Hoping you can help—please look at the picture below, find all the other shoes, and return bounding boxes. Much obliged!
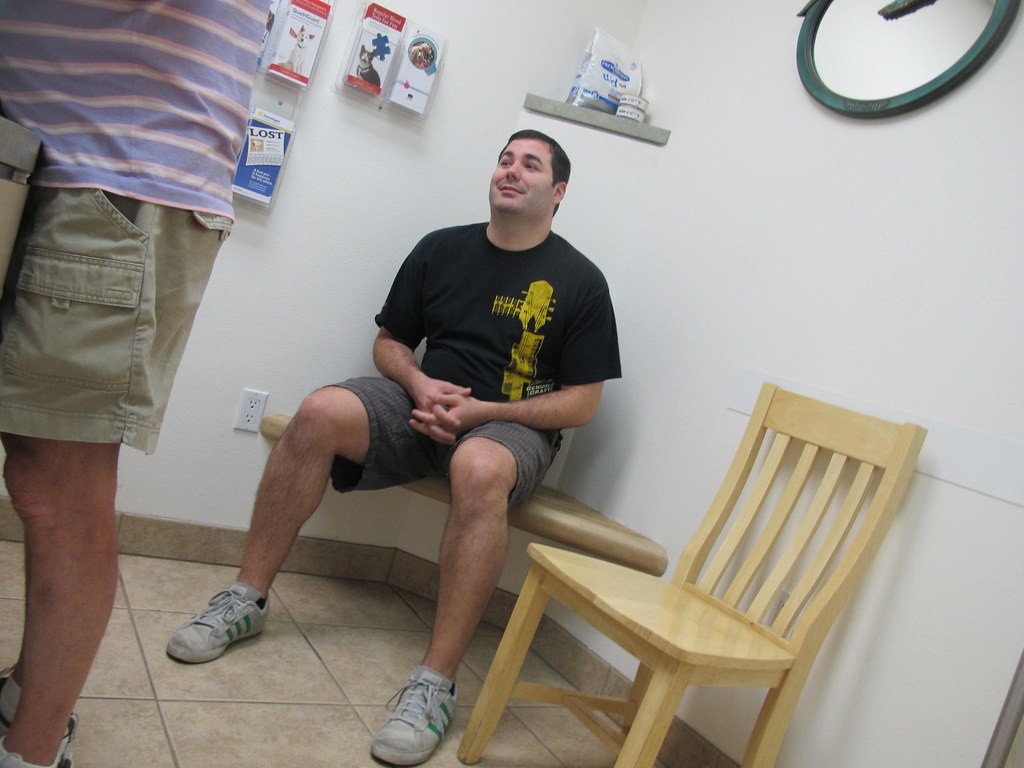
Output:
[0,664,78,768]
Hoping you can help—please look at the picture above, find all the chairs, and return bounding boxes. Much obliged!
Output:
[457,380,928,768]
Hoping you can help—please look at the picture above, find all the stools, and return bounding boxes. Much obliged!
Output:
[260,413,668,578]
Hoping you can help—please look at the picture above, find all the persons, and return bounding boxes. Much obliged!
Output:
[0,0,273,768]
[166,129,623,766]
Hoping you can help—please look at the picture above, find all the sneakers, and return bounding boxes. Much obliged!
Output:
[167,582,270,664]
[371,664,457,766]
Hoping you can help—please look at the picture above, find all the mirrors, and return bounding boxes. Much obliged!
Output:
[795,0,1021,120]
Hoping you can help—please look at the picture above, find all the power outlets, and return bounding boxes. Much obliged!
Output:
[233,388,269,433]
